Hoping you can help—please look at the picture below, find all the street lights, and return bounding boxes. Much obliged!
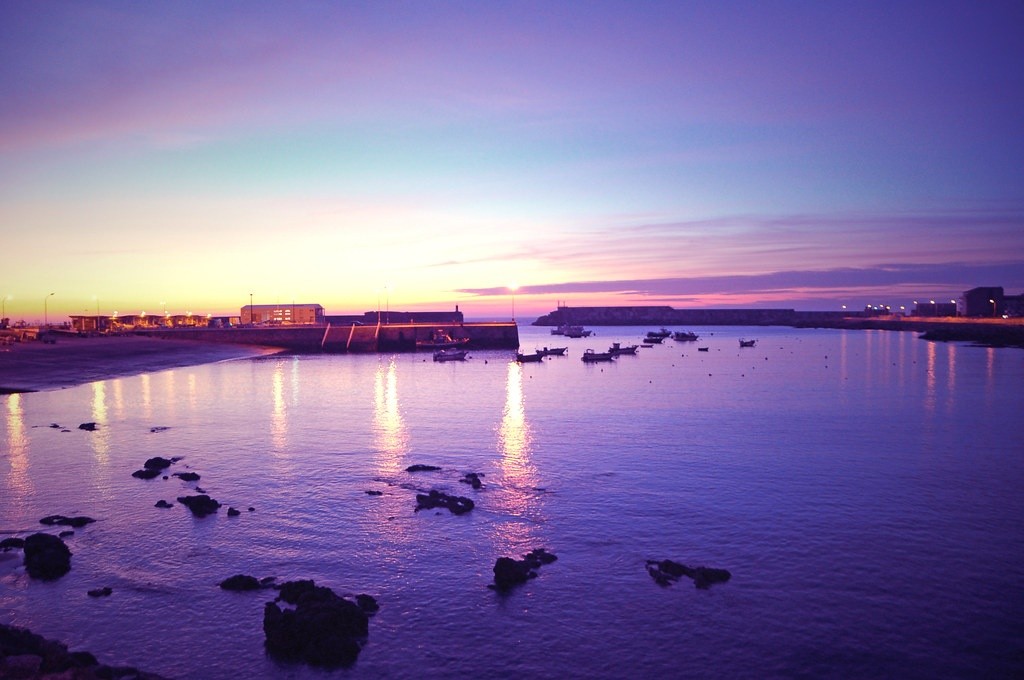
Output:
[250,294,253,323]
[44,292,56,325]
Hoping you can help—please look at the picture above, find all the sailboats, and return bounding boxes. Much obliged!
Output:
[550,301,584,335]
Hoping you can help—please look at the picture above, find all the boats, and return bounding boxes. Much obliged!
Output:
[581,327,710,362]
[416,330,471,351]
[739,338,756,347]
[535,346,568,355]
[432,348,468,362]
[564,328,593,339]
[517,353,544,362]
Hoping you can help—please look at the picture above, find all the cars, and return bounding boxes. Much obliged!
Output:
[254,322,268,328]
[350,320,364,326]
